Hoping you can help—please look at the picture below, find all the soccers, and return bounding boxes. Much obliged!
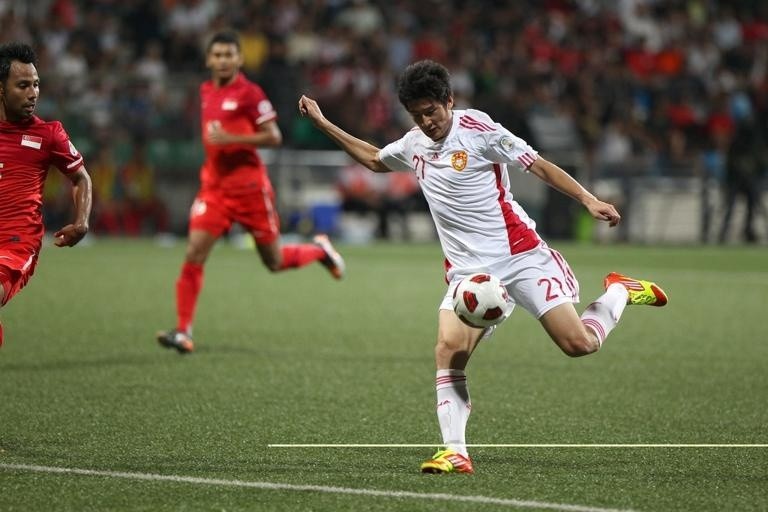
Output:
[453,273,508,328]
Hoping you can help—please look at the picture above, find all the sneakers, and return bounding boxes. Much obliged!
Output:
[420,448,474,473]
[602,270,668,308]
[157,328,195,354]
[313,233,347,280]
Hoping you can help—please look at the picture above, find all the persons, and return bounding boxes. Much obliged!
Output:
[154,31,347,353]
[297,59,669,473]
[0,42,93,346]
[1,0,766,248]
